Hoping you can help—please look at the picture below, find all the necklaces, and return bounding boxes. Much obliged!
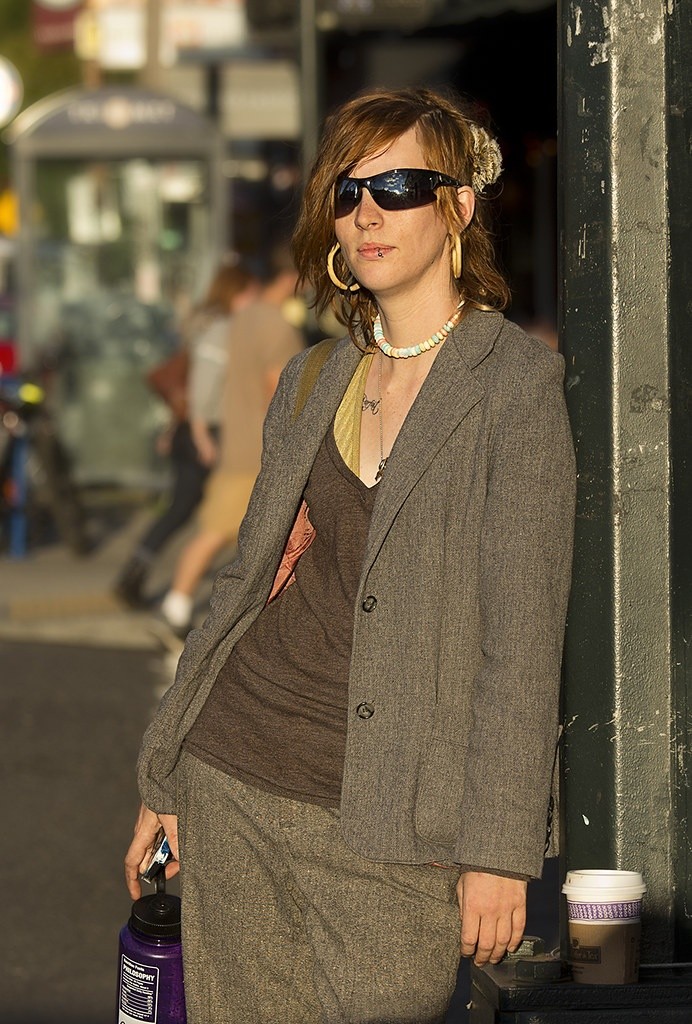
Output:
[372,304,462,358]
[374,351,389,481]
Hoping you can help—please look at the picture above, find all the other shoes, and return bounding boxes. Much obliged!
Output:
[107,580,163,613]
[153,605,196,654]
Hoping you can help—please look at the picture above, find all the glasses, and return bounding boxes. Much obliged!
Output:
[334,169,463,219]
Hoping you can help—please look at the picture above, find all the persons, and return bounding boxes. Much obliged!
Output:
[126,82,578,1024]
[102,246,308,648]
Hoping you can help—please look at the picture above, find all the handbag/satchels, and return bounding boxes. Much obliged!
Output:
[144,341,192,420]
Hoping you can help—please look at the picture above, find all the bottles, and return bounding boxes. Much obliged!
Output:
[117,862,187,1024]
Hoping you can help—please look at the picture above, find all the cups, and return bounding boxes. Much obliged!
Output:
[561,868,648,985]
